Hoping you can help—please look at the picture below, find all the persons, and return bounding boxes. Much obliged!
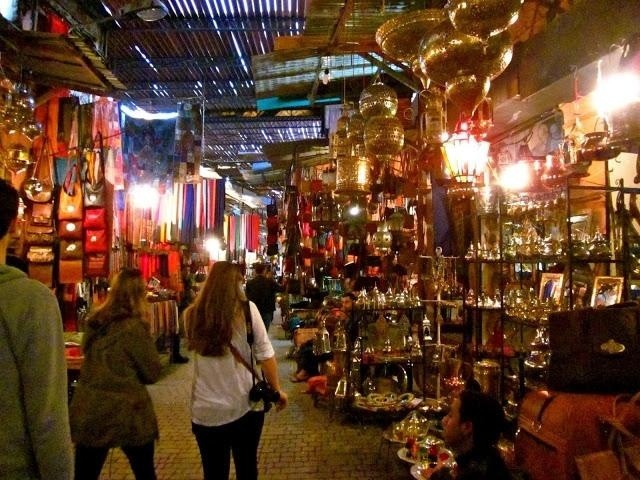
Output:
[65,266,163,479]
[0,177,75,480]
[246,263,288,333]
[288,292,357,382]
[168,260,193,367]
[440,387,517,480]
[180,260,287,480]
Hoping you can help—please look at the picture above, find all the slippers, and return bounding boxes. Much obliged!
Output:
[290,368,309,383]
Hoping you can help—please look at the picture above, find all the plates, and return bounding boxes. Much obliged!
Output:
[397,443,454,464]
[410,462,457,480]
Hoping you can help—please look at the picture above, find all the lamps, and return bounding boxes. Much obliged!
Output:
[412,89,454,147]
[67,0,168,34]
[338,153,371,193]
[419,22,515,116]
[358,71,399,115]
[445,0,548,56]
[360,112,405,169]
[329,113,361,157]
[376,8,449,64]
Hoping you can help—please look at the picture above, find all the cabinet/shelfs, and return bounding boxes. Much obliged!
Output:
[343,179,640,418]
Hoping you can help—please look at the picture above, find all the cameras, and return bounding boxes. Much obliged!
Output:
[250,380,280,405]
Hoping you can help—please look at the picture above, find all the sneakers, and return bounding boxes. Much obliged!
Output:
[170,356,189,364]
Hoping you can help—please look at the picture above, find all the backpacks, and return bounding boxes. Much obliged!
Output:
[59,260,84,285]
[512,388,605,480]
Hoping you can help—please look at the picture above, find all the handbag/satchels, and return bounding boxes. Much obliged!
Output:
[548,306,638,394]
[27,225,55,247]
[28,261,54,289]
[86,252,108,279]
[84,183,105,209]
[30,201,53,224]
[59,186,84,221]
[283,200,407,306]
[83,206,106,230]
[25,177,52,202]
[84,226,108,255]
[25,247,55,263]
[59,240,84,260]
[60,220,83,239]
